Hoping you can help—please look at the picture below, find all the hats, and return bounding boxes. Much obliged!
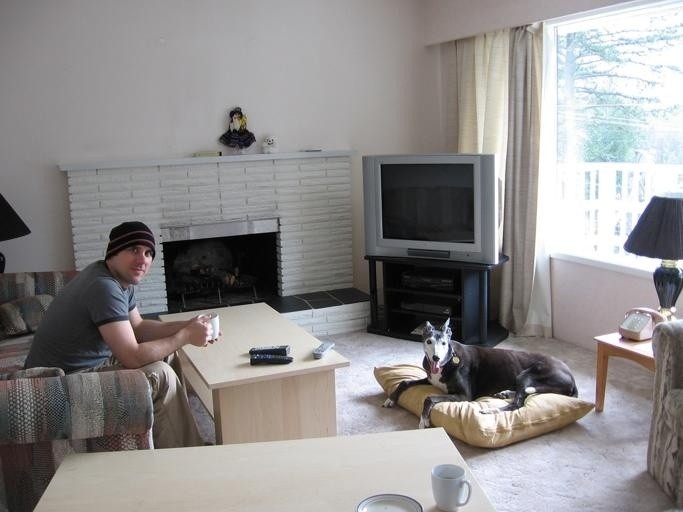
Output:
[105,221,156,263]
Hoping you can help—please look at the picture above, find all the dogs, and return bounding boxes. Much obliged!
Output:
[381,317,579,429]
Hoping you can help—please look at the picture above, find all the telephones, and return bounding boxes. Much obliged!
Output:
[618,307,668,342]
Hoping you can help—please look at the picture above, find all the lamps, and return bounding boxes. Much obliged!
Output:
[0,191,31,272]
[620,194,681,314]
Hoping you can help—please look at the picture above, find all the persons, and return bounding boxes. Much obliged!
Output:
[25,222,221,449]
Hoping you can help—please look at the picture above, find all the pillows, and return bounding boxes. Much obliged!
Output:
[372,358,595,448]
[1,366,65,379]
[0,292,56,338]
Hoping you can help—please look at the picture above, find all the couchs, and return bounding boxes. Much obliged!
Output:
[647,319,683,511]
[0,270,153,510]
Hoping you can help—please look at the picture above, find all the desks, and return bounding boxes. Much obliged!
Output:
[592,314,654,412]
[33,425,494,510]
[153,301,351,444]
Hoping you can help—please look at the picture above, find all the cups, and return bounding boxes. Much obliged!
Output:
[198,312,220,340]
[431,463,472,510]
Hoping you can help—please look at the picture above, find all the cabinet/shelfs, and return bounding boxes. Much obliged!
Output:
[365,258,511,350]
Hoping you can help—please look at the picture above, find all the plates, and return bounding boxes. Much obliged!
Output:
[357,494,425,512]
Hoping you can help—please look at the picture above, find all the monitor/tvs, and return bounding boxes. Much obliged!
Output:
[361,152,502,266]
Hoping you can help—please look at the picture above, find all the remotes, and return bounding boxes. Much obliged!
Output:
[250,353,293,365]
[312,341,335,359]
[249,344,290,356]
